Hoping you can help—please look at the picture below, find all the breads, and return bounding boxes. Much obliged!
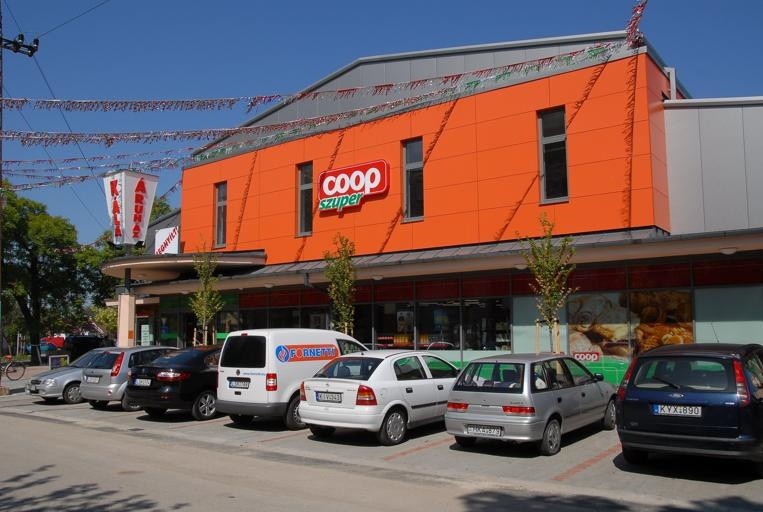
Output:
[568,294,641,357]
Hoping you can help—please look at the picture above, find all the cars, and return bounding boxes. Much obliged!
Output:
[25,347,116,405]
[122,343,223,421]
[297,349,488,446]
[79,346,181,412]
[445,352,620,456]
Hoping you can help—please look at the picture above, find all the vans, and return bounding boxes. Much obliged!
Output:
[214,328,375,431]
[614,343,763,475]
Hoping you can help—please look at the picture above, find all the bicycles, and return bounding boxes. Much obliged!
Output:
[0,354,28,382]
